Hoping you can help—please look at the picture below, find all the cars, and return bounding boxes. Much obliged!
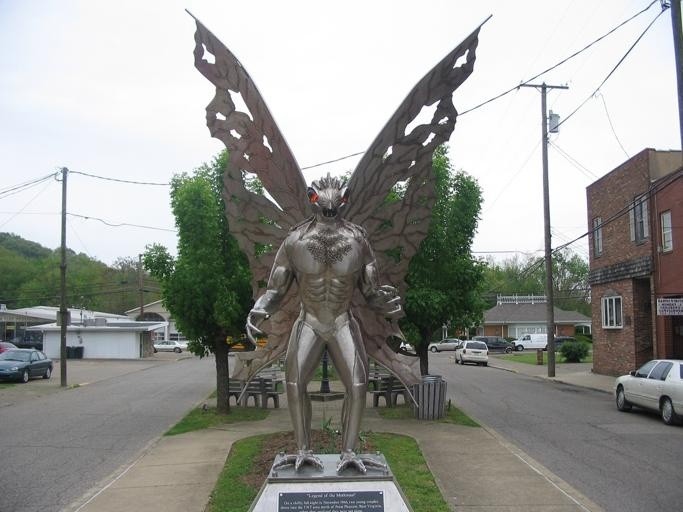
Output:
[0,347,51,382]
[400,342,418,357]
[427,337,463,353]
[0,340,16,357]
[551,335,581,344]
[9,337,43,351]
[153,339,189,354]
[455,337,488,366]
[615,358,682,424]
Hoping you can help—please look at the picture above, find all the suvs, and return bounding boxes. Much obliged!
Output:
[472,335,515,355]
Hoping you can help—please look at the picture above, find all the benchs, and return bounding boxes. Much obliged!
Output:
[370,376,413,407]
[229,378,282,409]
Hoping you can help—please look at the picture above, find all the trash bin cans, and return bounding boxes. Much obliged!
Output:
[537,348,543,365]
[65,346,84,359]
[413,374,447,420]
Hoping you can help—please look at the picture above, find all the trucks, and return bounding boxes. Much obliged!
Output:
[511,333,556,352]
